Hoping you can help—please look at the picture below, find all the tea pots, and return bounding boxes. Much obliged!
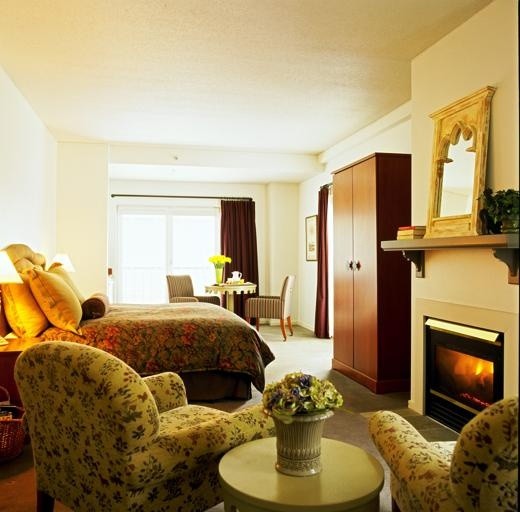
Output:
[232,271,243,280]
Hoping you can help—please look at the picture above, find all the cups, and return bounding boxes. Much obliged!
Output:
[226,277,245,286]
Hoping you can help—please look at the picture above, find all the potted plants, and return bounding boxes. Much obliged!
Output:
[476,186,520,233]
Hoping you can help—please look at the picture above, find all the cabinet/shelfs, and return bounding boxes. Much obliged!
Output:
[331,152,410,393]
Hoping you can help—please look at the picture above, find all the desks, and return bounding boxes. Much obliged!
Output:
[203,281,257,312]
[216,434,385,512]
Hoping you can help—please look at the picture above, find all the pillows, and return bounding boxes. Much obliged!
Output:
[1,244,111,341]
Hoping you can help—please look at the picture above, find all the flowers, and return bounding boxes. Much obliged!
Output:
[263,368,346,425]
[208,255,232,269]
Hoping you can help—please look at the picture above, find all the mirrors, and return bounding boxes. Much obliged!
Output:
[424,86,498,238]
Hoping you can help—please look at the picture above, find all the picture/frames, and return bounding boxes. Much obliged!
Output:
[305,215,319,262]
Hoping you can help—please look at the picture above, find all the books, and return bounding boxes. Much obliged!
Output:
[396,225,426,240]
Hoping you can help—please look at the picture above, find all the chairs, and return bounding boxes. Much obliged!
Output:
[369,394,519,511]
[166,273,221,306]
[245,274,295,341]
[13,341,276,512]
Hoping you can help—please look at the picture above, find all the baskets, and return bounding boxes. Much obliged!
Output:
[0,405,26,464]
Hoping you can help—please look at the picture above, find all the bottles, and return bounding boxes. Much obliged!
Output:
[107,268,115,305]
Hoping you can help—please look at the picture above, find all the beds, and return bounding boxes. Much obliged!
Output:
[0,280,275,403]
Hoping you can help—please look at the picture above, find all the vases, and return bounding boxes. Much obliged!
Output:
[214,264,224,282]
[269,408,334,478]
